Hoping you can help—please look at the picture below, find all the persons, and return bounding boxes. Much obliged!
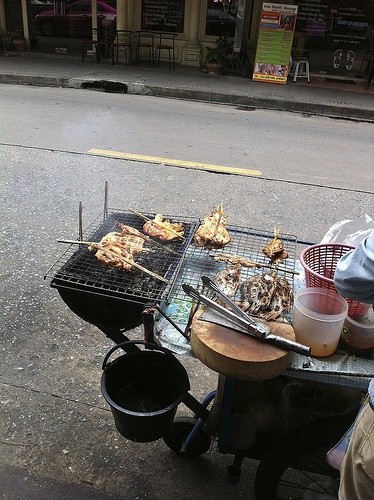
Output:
[333,228,373,500]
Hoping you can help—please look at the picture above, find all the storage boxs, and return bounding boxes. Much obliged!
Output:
[304,29,326,38]
[304,19,327,30]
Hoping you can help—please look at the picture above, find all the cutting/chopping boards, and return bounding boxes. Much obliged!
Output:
[189,300,296,383]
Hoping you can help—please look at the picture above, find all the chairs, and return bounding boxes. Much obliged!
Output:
[81,27,100,65]
[154,32,178,72]
[134,30,155,72]
[110,29,134,68]
[96,28,116,66]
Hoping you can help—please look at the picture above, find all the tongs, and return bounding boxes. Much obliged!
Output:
[182,274,267,338]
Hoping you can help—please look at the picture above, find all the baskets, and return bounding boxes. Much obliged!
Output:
[300,243,371,317]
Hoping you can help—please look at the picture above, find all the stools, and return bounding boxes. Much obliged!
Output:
[291,57,310,83]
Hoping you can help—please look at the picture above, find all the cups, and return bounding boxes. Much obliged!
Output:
[343,303,374,350]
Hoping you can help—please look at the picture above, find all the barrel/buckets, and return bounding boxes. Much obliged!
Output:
[293,288,349,357]
[101,340,190,444]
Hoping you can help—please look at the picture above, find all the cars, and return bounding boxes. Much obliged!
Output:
[32,0,117,37]
[160,8,237,37]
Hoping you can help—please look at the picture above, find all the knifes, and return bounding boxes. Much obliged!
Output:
[197,305,311,358]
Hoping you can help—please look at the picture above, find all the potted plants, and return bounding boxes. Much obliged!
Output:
[29,31,40,50]
[205,35,234,76]
[11,30,27,50]
